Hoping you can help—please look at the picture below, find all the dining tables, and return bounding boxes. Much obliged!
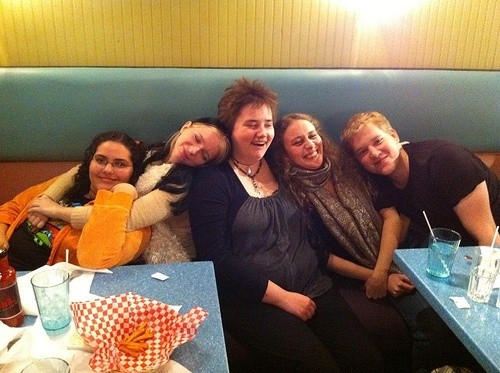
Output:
[14,262,230,373]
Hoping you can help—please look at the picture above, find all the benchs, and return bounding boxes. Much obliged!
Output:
[0,67,500,280]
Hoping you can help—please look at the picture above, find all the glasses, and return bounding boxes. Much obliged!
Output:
[92,153,134,169]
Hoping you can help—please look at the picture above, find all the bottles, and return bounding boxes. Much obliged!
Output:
[0,247,25,327]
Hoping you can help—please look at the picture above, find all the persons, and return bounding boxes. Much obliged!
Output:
[0,77,500,373]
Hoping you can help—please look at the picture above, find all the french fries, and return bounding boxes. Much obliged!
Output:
[116,323,154,357]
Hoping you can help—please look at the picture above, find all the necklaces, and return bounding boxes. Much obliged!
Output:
[230,155,263,188]
[233,157,258,173]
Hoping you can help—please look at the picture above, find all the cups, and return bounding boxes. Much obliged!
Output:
[468,246,500,304]
[21,357,70,373]
[426,227,461,278]
[30,268,72,335]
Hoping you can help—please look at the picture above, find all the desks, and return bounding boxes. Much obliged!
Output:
[393,246,500,373]
